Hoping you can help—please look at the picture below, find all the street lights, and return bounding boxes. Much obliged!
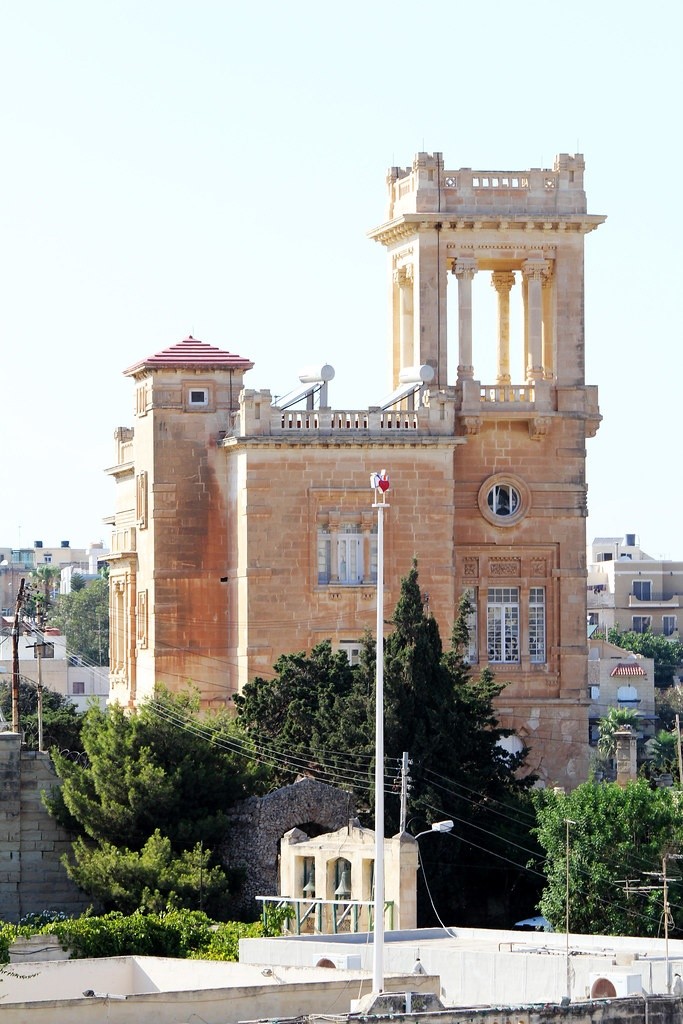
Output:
[350,467,391,1012]
[0,665,44,752]
